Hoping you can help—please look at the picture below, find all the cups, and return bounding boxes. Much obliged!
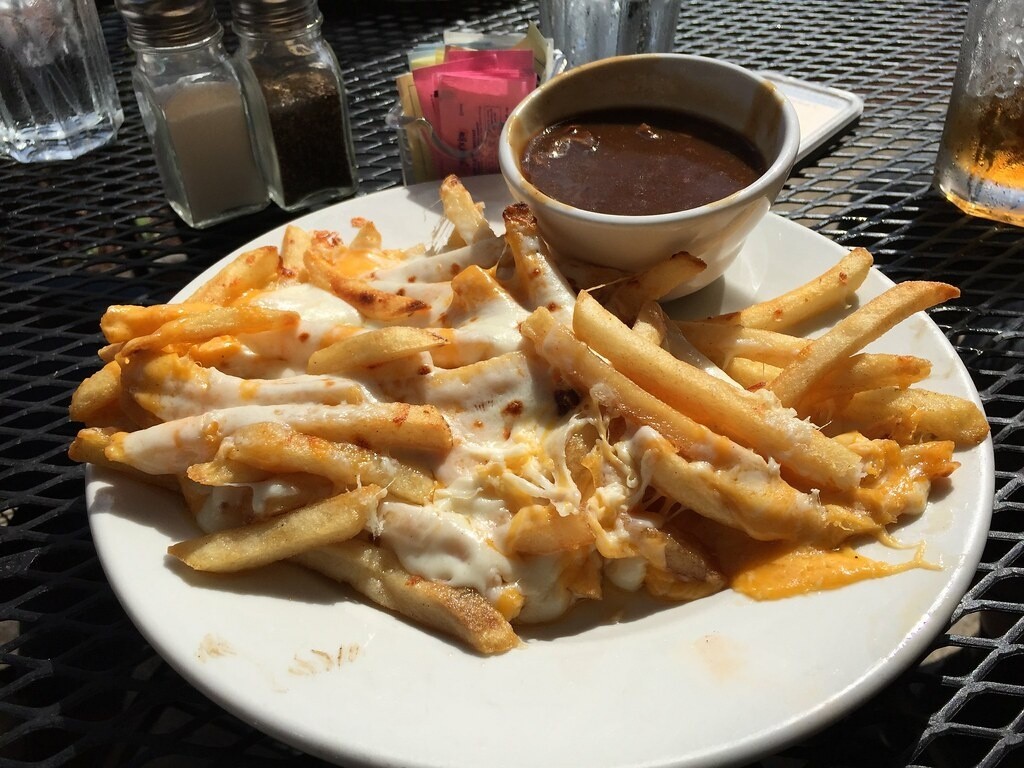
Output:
[934,0,1024,228]
[1,0,125,163]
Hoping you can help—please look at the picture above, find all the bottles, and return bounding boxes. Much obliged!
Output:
[230,0,357,213]
[112,0,272,226]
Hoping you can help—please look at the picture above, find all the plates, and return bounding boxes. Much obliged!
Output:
[85,170,991,768]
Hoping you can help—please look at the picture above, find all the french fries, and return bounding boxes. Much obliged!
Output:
[69,170,988,659]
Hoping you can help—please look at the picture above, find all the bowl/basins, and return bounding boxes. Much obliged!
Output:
[500,53,800,300]
